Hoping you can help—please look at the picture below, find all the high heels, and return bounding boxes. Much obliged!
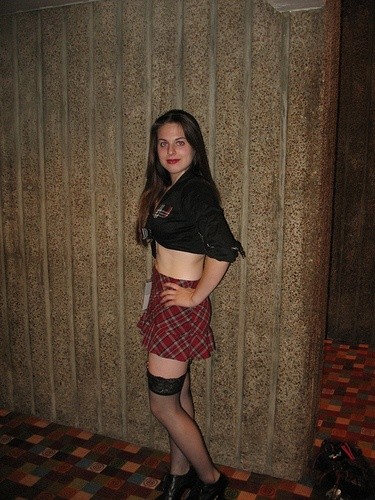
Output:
[155,467,197,500]
[187,474,229,499]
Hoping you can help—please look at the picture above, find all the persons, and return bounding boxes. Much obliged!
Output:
[134,110,247,499]
